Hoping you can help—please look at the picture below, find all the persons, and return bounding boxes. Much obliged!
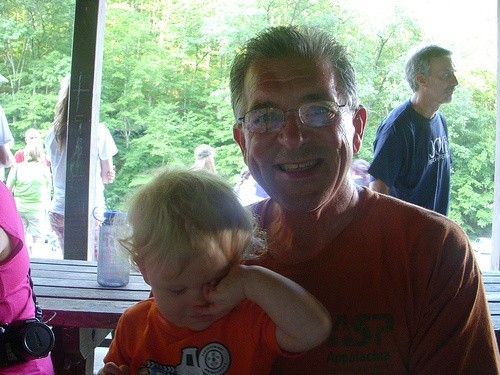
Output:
[0,72,117,374]
[368,46,459,215]
[101,169,333,375]
[226,26,500,375]
[190,144,216,173]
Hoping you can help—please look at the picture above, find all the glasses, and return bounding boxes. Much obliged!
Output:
[237,100,353,134]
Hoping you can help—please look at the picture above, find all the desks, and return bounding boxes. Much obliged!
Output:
[29,258,500,375]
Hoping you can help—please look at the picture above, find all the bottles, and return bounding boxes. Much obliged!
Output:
[93,205,132,287]
